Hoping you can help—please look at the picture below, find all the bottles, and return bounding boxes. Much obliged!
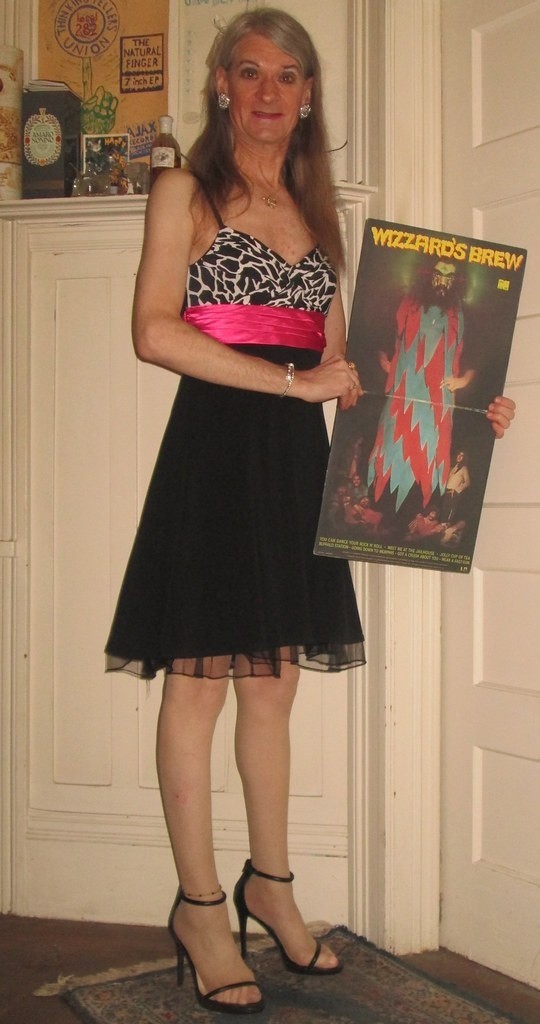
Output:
[151,116,181,194]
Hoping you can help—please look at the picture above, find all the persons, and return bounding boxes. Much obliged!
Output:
[106,8,515,1014]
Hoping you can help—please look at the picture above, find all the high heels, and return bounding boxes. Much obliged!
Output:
[233,859,342,975]
[168,885,266,1014]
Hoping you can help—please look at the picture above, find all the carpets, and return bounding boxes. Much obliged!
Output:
[34,921,531,1024]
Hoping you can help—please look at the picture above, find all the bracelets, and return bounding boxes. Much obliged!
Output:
[280,362,295,398]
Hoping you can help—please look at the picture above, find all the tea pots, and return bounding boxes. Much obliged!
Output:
[69,158,110,196]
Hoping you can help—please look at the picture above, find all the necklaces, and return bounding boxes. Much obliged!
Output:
[252,191,280,208]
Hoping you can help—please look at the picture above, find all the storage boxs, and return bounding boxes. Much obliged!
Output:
[21,91,81,199]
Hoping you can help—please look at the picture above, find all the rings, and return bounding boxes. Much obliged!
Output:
[347,360,355,370]
